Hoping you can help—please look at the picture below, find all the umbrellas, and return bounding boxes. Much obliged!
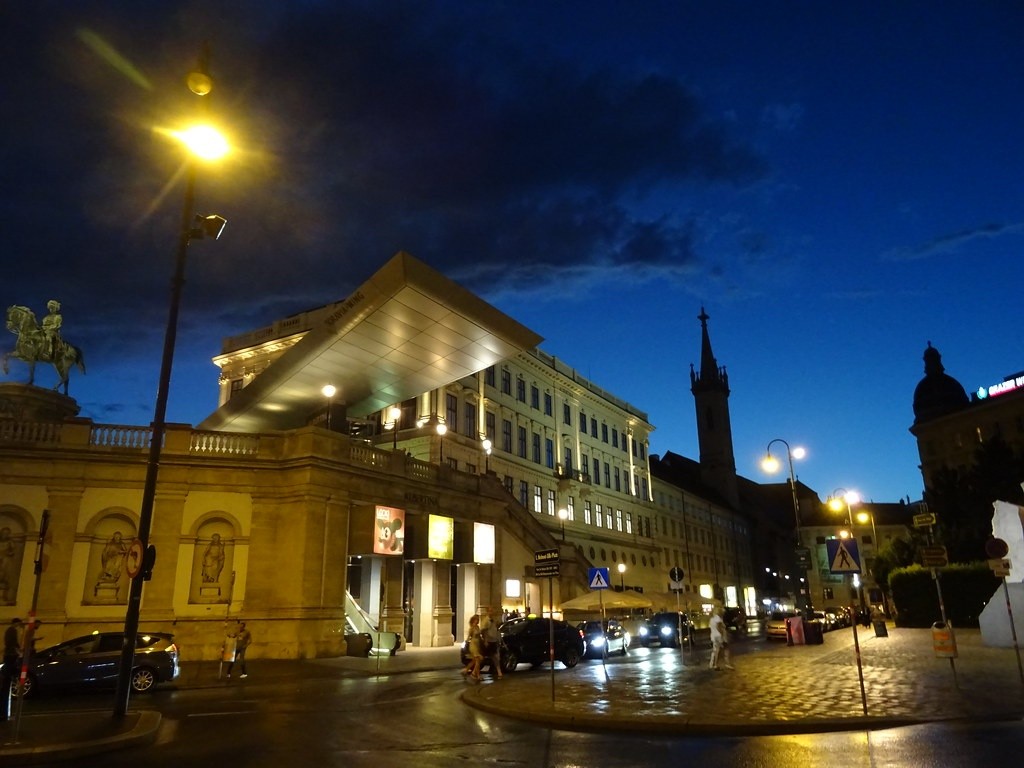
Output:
[557,588,721,655]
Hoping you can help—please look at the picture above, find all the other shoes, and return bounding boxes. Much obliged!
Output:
[714,667,722,671]
[498,673,503,676]
[460,670,466,680]
[239,674,247,679]
[726,665,734,670]
[226,674,230,676]
[470,673,477,678]
[475,677,484,681]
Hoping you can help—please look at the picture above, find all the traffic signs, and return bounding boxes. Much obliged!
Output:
[913,512,936,526]
[921,556,948,568]
[920,545,947,557]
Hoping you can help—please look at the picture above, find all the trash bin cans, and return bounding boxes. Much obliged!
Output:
[803,622,824,644]
[873,620,887,636]
[931,621,958,658]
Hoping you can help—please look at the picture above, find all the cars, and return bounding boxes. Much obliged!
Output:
[639,611,696,647]
[765,608,802,640]
[577,620,633,659]
[804,602,886,633]
[0,632,182,700]
[461,616,587,673]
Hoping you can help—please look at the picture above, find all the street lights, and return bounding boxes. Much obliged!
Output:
[761,439,815,622]
[854,511,886,614]
[109,44,229,736]
[828,487,868,623]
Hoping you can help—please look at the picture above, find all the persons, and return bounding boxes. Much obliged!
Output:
[861,604,896,629]
[41,300,62,357]
[648,608,734,671]
[0,527,16,604]
[99,531,128,582]
[4,618,21,659]
[201,533,225,583]
[225,623,252,679]
[26,620,44,655]
[459,607,531,681]
